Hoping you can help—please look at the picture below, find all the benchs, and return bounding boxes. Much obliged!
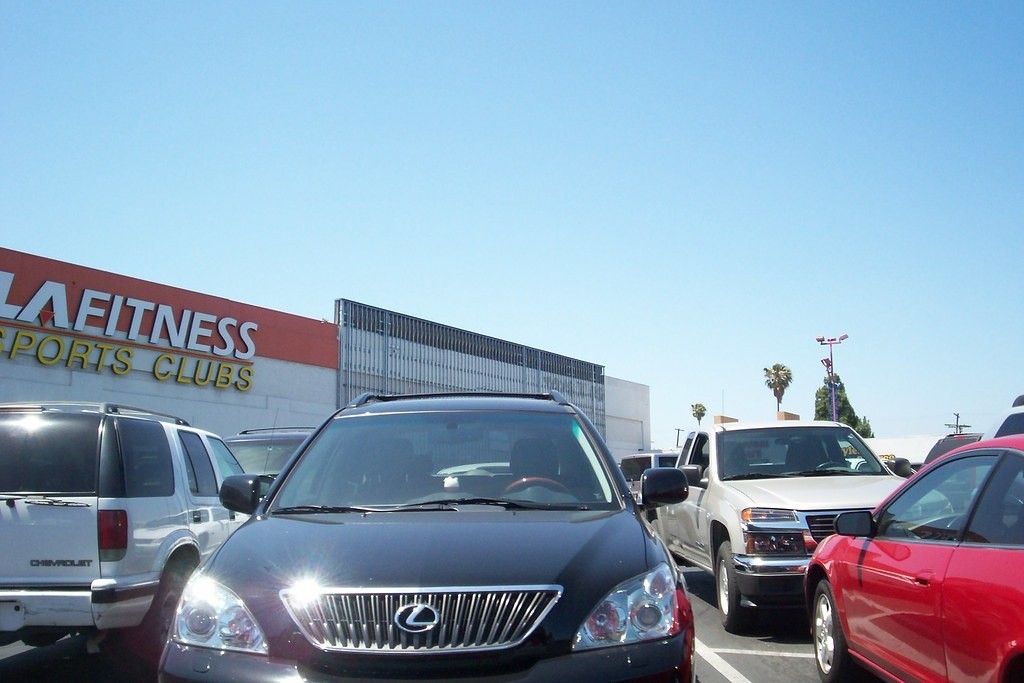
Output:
[432,474,512,497]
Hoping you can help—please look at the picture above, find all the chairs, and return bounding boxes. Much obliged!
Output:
[783,436,828,472]
[356,434,422,503]
[723,441,751,477]
[509,436,561,490]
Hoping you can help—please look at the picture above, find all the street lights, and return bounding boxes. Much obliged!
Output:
[816,332,849,420]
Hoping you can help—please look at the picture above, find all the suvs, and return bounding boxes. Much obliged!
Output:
[221,427,318,475]
[0,403,247,683]
[158,389,1024,683]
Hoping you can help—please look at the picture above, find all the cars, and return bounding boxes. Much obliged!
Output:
[804,434,1023,682]
